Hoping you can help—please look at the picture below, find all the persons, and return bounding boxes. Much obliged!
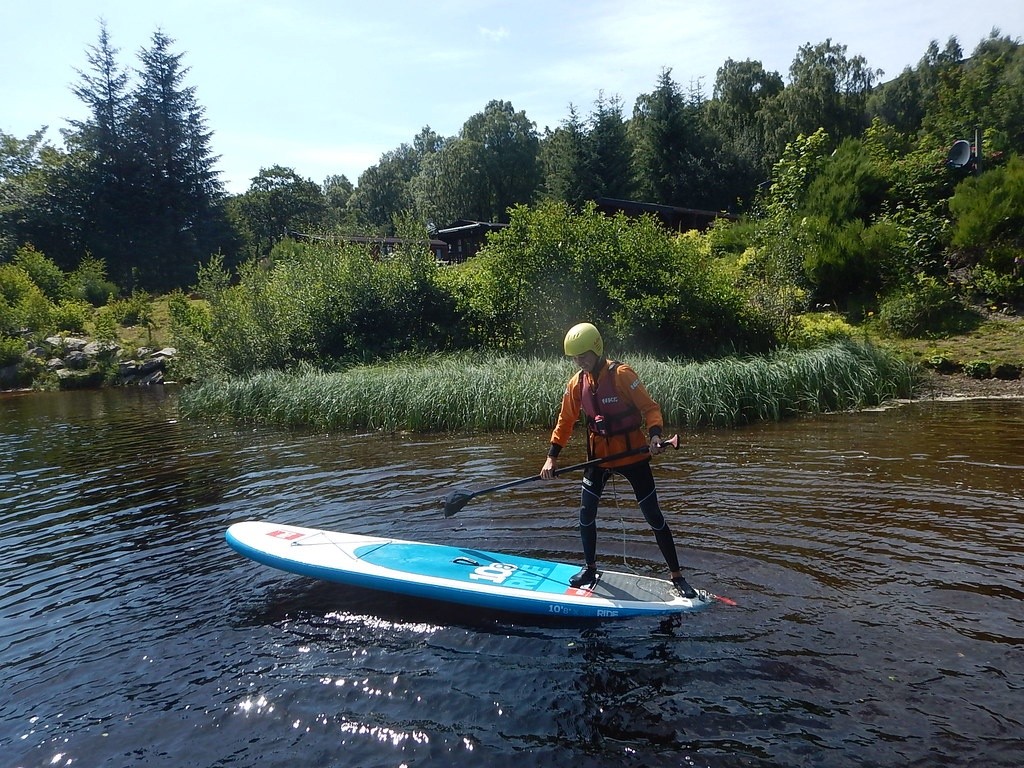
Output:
[539,322,698,598]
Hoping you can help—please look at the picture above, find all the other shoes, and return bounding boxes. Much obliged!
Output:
[671,577,695,598]
[570,566,597,586]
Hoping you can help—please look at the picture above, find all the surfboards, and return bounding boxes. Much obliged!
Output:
[224,518,712,615]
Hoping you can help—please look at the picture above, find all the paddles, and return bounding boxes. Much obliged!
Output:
[444,434,680,516]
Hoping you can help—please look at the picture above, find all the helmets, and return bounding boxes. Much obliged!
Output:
[564,323,603,358]
